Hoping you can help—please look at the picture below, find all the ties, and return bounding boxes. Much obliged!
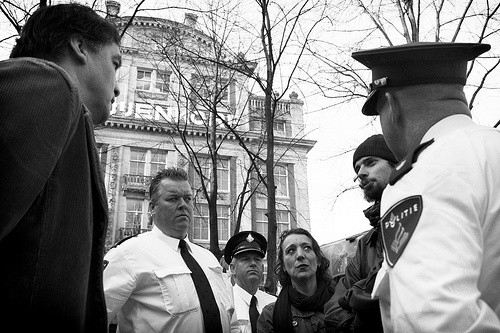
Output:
[249,295,260,332]
[177,239,223,333]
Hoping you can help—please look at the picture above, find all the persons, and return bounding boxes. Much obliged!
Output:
[351,41,500,333]
[103,167,242,333]
[257,227,336,333]
[324,132,400,333]
[0,2,122,333]
[224,230,278,332]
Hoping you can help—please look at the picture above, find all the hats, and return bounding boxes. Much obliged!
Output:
[351,42,491,116]
[224,230,267,264]
[353,134,398,167]
